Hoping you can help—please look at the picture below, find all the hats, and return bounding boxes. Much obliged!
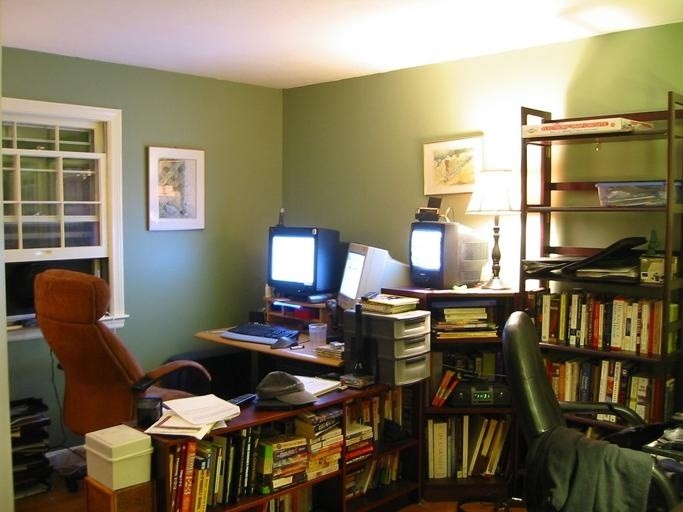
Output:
[256,371,320,405]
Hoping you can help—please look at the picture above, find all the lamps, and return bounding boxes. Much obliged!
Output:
[464,167,520,289]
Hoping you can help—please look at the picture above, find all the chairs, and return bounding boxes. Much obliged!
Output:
[33,268,212,434]
[500,311,682,512]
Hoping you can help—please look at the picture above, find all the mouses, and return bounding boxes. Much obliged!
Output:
[270,337,296,349]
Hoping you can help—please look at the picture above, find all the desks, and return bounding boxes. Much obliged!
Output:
[191,296,347,369]
[641,421,682,460]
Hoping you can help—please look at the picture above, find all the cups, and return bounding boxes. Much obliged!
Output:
[309,322,328,351]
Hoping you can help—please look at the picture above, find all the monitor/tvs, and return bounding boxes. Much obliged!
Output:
[411,221,492,288]
[338,240,409,306]
[265,225,348,298]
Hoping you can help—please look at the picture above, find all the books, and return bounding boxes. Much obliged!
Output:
[315,344,344,358]
[528,288,682,427]
[162,373,415,510]
[357,293,420,314]
[424,298,511,479]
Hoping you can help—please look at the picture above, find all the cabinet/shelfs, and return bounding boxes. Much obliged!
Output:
[381,287,547,503]
[520,91,682,444]
[135,373,423,512]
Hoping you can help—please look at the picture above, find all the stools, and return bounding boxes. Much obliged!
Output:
[82,476,157,512]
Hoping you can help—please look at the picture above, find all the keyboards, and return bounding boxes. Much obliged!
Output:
[219,320,300,346]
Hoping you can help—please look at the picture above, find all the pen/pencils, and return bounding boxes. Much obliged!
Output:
[290,345,304,350]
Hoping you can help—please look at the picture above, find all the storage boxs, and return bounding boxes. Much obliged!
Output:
[343,308,431,385]
[85,424,154,491]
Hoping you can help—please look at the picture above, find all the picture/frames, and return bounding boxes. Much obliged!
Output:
[422,131,484,196]
[146,144,207,232]
